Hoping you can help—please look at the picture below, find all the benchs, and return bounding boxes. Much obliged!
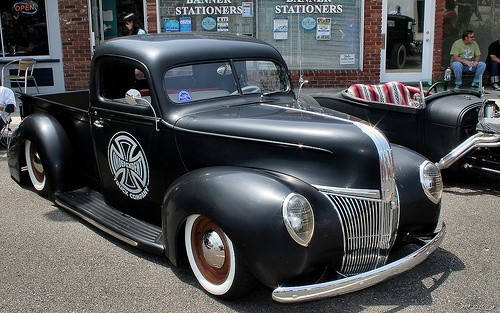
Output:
[348,81,414,107]
[140,87,220,95]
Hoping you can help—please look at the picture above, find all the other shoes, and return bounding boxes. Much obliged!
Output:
[471,82,484,89]
[455,84,461,89]
[491,83,500,90]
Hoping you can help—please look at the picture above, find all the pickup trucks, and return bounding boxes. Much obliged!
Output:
[7,32,446,302]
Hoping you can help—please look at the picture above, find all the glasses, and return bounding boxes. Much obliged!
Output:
[467,36,474,38]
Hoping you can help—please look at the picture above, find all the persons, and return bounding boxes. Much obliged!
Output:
[25,24,42,55]
[485,39,500,91]
[450,30,486,90]
[0,86,17,131]
[121,11,146,79]
[389,5,402,15]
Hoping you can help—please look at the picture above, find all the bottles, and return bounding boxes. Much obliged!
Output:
[444,67,452,81]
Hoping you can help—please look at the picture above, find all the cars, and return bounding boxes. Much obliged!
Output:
[307,80,500,177]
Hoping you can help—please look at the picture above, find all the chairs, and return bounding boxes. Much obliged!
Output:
[0,113,11,147]
[2,57,40,95]
